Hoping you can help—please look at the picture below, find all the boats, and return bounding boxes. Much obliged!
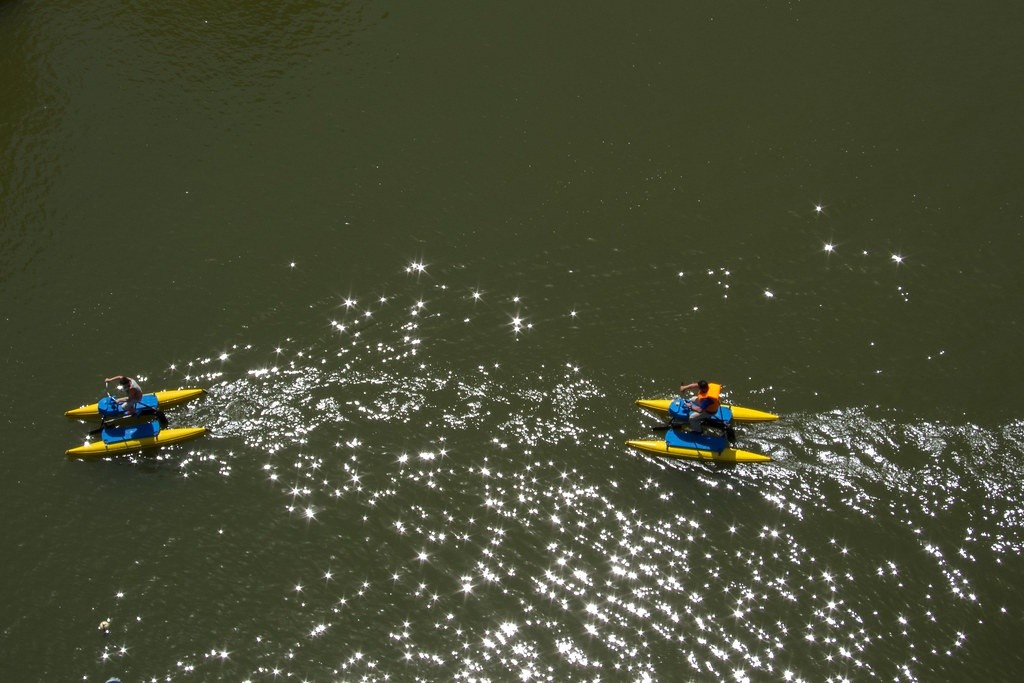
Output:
[624,382,784,464]
[64,378,210,458]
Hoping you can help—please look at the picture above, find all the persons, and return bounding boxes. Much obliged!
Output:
[681,380,721,432]
[105,376,142,411]
